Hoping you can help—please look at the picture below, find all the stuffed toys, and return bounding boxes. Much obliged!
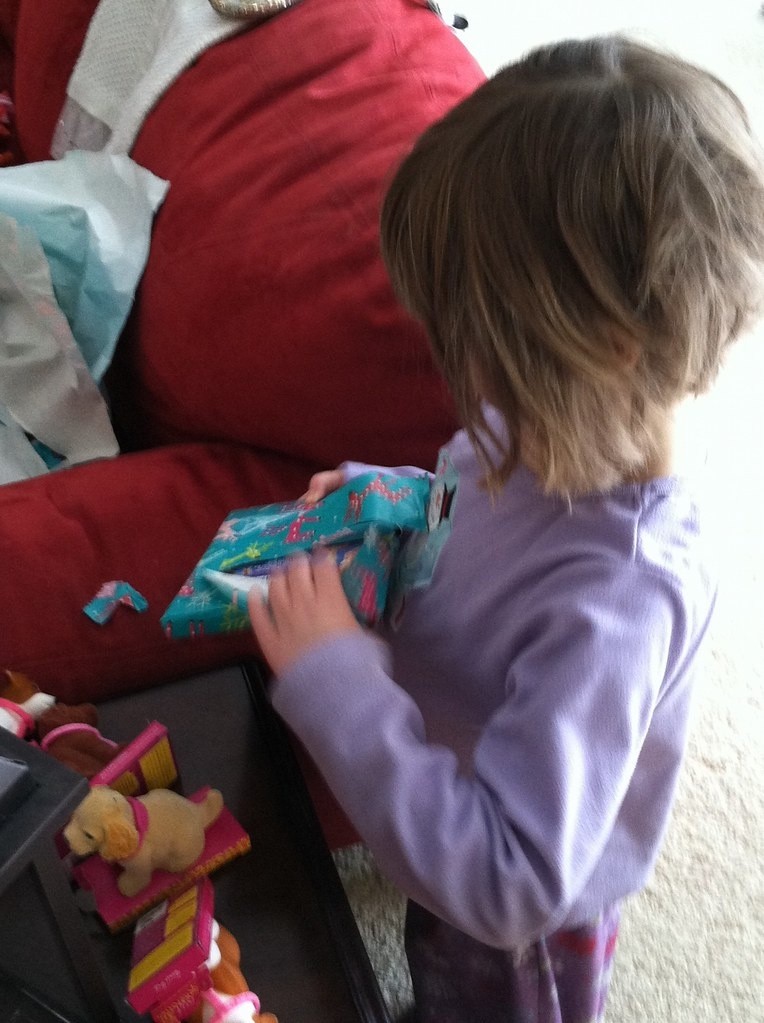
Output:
[202,919,278,1023]
[0,670,101,777]
[65,785,225,893]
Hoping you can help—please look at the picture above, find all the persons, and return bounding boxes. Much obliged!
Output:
[247,38,764,1023]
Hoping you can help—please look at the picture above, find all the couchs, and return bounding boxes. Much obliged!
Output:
[0,0,482,711]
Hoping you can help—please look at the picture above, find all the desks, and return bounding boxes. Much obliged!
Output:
[0,664,392,1023]
